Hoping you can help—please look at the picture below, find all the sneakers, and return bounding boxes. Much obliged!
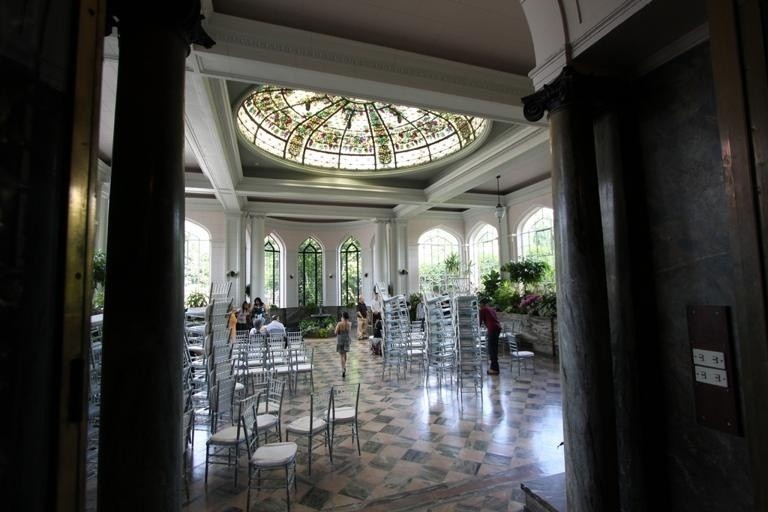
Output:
[487,369,498,374]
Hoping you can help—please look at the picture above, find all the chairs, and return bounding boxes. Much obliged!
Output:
[369,280,521,401]
[505,332,536,375]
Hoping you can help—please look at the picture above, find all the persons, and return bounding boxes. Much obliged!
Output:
[333,311,353,378]
[439,293,451,316]
[478,298,502,375]
[399,294,412,314]
[371,292,381,328]
[416,297,426,333]
[356,295,370,341]
[226,296,288,350]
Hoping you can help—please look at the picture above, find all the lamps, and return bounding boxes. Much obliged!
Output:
[494,176,506,225]
